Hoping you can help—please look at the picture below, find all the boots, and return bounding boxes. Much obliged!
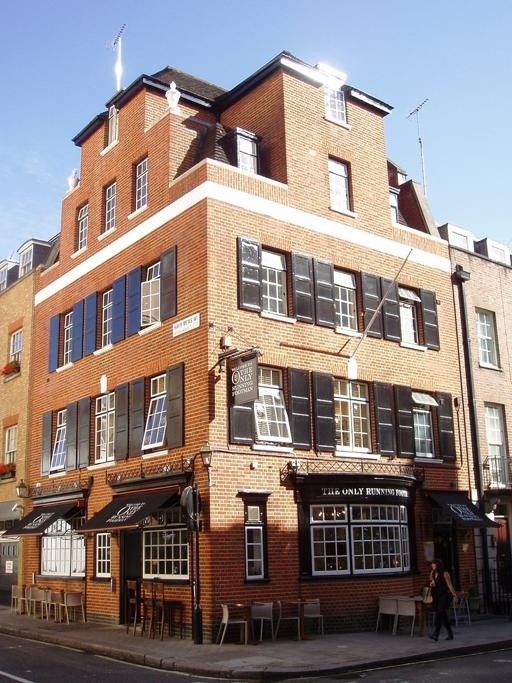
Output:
[428,632,454,641]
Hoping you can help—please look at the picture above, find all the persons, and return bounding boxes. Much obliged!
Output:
[429,559,457,641]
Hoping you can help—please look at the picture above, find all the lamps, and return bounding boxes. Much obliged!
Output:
[16,478,28,498]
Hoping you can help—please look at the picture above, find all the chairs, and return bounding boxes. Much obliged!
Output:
[126,579,183,640]
[11,584,86,625]
[214,598,324,646]
[375,594,471,638]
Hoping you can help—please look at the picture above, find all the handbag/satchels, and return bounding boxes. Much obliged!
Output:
[423,587,433,603]
